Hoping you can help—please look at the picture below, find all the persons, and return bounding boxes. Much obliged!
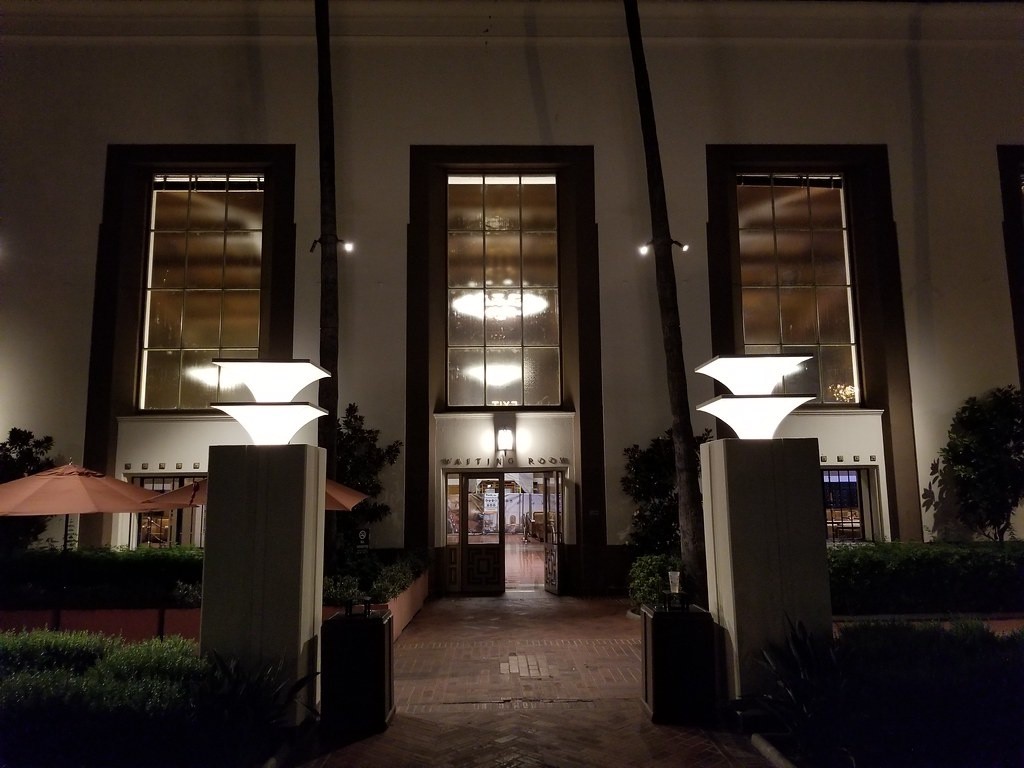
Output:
[522,511,531,534]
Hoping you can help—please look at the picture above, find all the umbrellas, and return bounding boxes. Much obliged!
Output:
[0,458,199,550]
[140,478,370,512]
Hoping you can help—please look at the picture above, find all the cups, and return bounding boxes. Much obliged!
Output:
[669,571,680,593]
